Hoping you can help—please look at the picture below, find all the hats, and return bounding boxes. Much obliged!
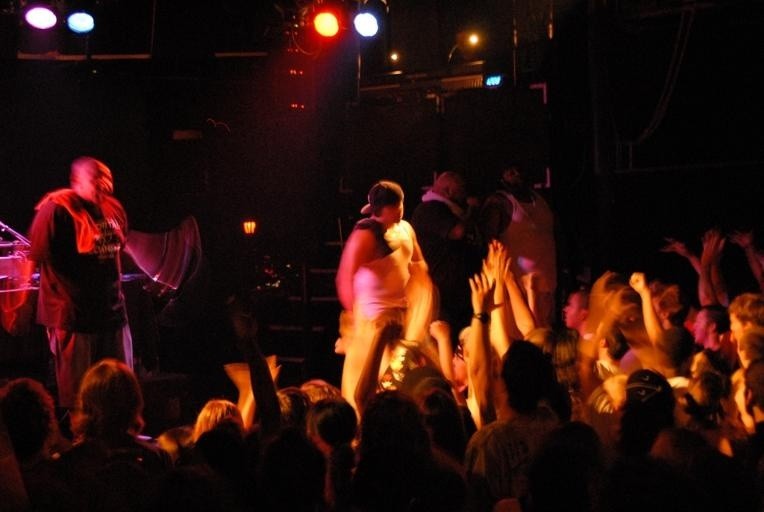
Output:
[360,181,404,215]
